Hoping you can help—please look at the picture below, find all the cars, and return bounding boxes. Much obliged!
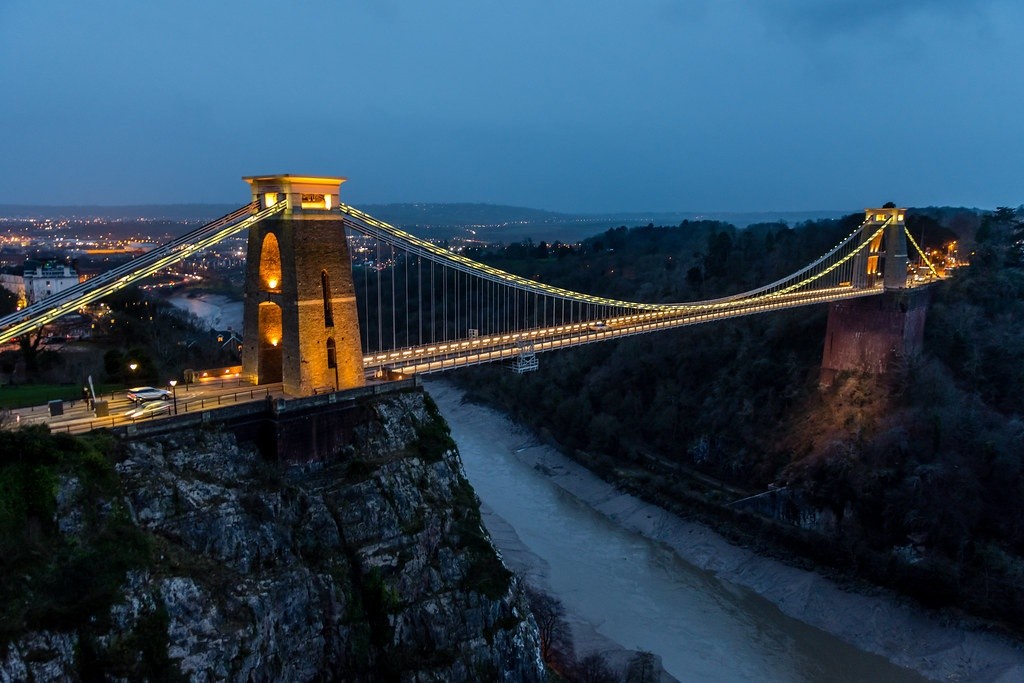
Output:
[585,324,612,331]
[126,400,172,420]
[128,387,174,401]
